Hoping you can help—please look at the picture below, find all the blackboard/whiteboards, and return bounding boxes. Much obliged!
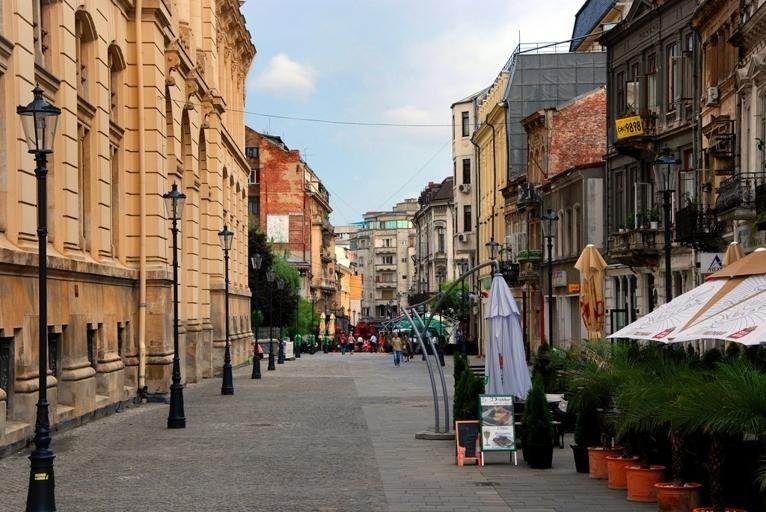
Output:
[456,420,481,460]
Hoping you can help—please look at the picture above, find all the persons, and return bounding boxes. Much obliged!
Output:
[346,334,356,355]
[338,333,347,355]
[369,333,377,353]
[400,335,413,363]
[391,331,402,366]
[377,331,385,352]
[356,335,363,352]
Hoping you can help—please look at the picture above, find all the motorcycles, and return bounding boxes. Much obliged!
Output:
[297,342,318,355]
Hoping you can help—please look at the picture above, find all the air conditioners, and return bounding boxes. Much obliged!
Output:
[457,184,468,193]
[457,233,467,242]
[705,87,718,106]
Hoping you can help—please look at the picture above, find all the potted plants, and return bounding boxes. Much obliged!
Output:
[618,208,659,232]
[551,339,766,512]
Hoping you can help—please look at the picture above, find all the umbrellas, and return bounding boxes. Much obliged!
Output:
[604,245,766,346]
[317,311,326,355]
[578,243,609,340]
[722,240,745,268]
[483,273,534,404]
[328,313,336,353]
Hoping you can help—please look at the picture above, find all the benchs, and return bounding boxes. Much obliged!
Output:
[513,400,565,450]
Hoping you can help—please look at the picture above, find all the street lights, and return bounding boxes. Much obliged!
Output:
[217,222,233,396]
[420,278,427,362]
[537,209,558,348]
[456,257,467,360]
[266,268,276,371]
[249,250,262,381]
[651,143,681,303]
[434,270,443,338]
[162,181,186,429]
[485,237,498,286]
[14,79,60,512]
[295,287,301,356]
[309,293,361,336]
[386,285,416,321]
[276,278,285,365]
